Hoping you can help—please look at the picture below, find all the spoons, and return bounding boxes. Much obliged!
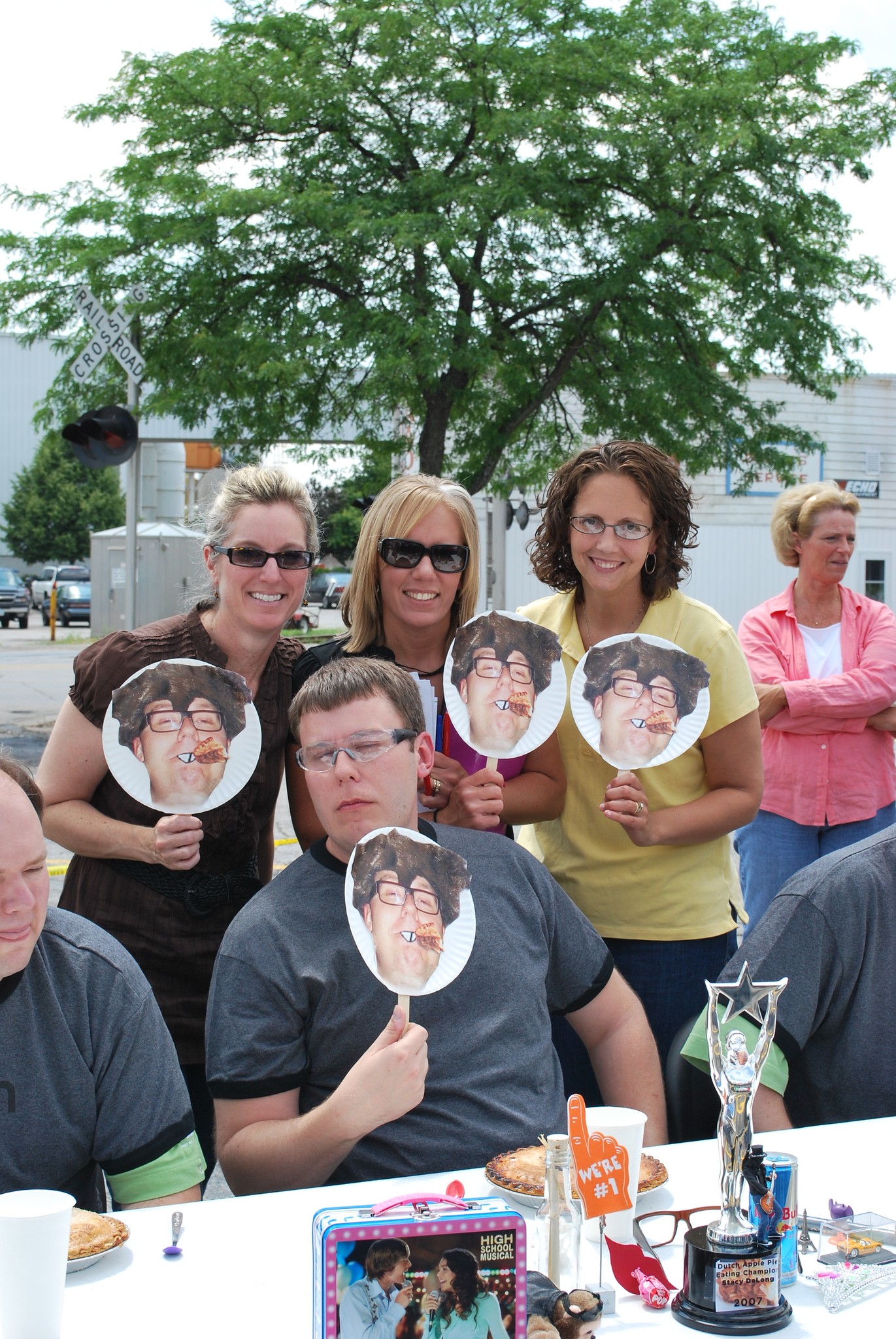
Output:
[162,1212,183,1255]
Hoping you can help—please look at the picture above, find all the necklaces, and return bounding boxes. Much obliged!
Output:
[583,599,647,647]
[794,592,841,626]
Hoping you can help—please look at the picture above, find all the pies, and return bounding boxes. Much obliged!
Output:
[192,737,230,763]
[508,692,534,719]
[66,1208,130,1275]
[484,1145,668,1213]
[415,922,444,953]
[644,710,677,735]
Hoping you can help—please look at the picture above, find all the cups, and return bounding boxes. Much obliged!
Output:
[584,1105,648,1243]
[0,1189,76,1339]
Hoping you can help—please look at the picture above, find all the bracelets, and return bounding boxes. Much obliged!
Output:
[433,809,439,824]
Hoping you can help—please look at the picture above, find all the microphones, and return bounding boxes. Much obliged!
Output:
[403,1280,416,1315]
[429,1290,439,1332]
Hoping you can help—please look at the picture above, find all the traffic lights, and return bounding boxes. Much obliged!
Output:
[353,495,378,515]
[505,502,533,531]
[63,405,137,469]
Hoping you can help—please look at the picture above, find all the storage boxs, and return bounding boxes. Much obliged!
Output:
[313,1193,527,1339]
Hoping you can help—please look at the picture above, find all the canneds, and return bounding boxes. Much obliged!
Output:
[748,1152,798,1288]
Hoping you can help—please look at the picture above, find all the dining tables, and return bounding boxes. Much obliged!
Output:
[0,1116,896,1339]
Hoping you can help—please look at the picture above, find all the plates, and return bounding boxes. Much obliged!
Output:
[484,1171,670,1213]
[65,1214,131,1273]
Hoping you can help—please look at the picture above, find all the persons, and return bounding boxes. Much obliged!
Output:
[280,475,566,855]
[338,1238,414,1339]
[742,1145,785,1246]
[205,658,670,1198]
[1,756,208,1212]
[421,1248,511,1339]
[682,822,896,1132]
[739,481,895,944]
[31,466,322,1194]
[509,441,750,1143]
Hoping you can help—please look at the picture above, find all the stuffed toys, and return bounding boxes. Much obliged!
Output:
[526,1270,603,1339]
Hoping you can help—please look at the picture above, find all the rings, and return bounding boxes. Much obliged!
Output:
[433,779,441,793]
[410,1295,412,1297]
[634,801,644,815]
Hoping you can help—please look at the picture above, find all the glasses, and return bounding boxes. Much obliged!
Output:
[632,1206,751,1278]
[377,538,470,574]
[369,880,442,915]
[142,711,227,733]
[466,657,536,684]
[603,677,680,708]
[213,544,315,570]
[569,516,654,540]
[296,728,420,773]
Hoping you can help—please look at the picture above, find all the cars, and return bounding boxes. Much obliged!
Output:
[303,572,355,609]
[41,584,91,628]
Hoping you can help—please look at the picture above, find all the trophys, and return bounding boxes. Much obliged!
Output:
[671,960,794,1336]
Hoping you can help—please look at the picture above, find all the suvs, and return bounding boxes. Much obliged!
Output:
[30,564,91,612]
[0,568,31,631]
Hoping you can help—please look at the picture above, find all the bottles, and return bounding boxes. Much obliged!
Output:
[534,1134,581,1294]
